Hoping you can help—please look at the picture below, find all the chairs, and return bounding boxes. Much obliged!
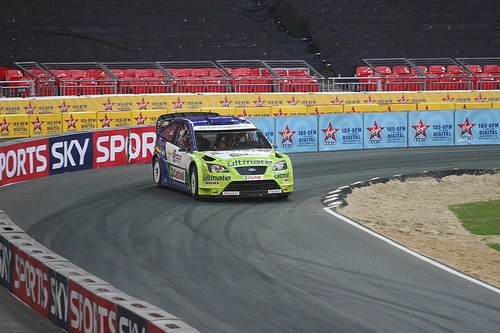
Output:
[0,67,319,97]
[356,65,500,91]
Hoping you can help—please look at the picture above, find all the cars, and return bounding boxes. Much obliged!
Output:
[152,112,294,202]
